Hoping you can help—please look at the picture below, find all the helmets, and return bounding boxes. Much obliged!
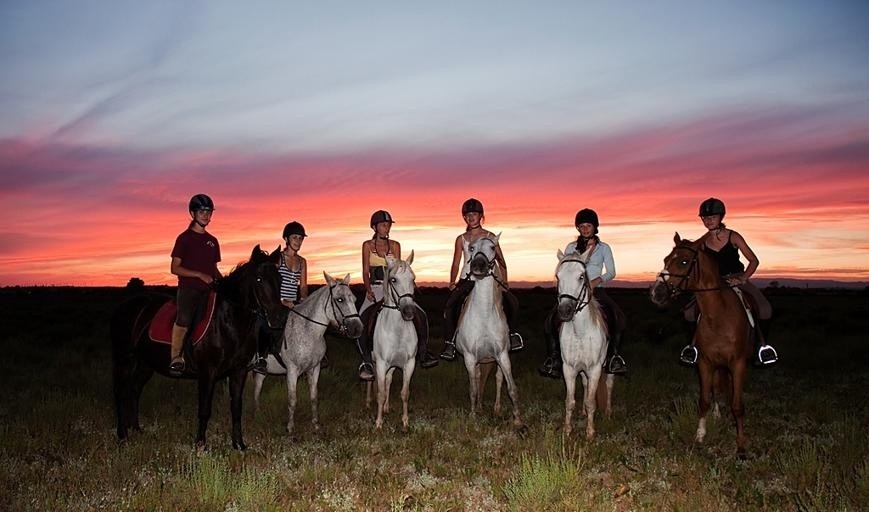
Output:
[284,221,308,239]
[462,198,483,215]
[370,210,395,227]
[189,193,216,214]
[699,197,725,217]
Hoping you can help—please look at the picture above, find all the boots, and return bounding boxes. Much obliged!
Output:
[170,322,189,370]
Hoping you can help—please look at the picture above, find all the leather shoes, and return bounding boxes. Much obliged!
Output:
[440,346,457,362]
[420,354,439,369]
[359,365,375,382]
[604,357,628,374]
[537,360,560,379]
[254,358,269,377]
[509,334,521,351]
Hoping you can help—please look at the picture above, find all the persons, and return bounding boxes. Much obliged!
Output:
[170,193,225,372]
[252,221,309,376]
[440,198,521,359]
[675,198,774,361]
[540,208,624,372]
[356,210,440,379]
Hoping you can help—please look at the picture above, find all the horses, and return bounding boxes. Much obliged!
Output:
[453,231,531,440]
[650,231,758,471]
[554,246,615,443]
[109,243,287,454]
[364,248,419,435]
[220,270,364,436]
